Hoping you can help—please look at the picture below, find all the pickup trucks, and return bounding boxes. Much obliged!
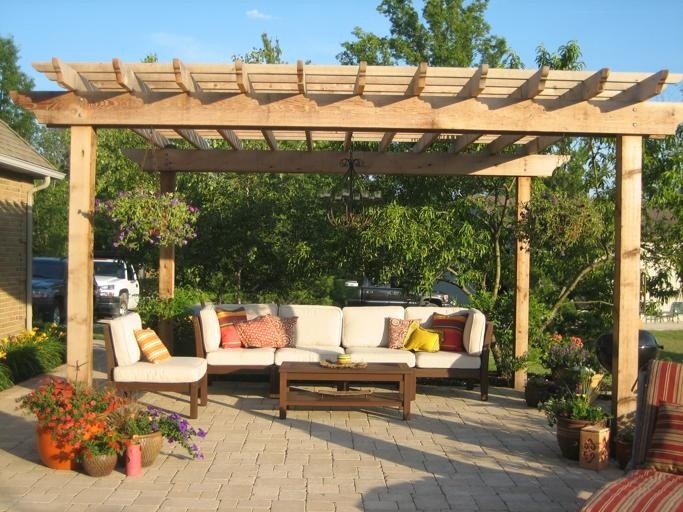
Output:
[331,268,449,307]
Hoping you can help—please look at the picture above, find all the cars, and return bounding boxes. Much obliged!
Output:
[32,255,104,329]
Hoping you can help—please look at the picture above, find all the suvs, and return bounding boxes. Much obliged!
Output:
[92,257,140,314]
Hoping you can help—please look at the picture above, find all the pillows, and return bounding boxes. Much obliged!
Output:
[387,312,469,351]
[215,307,298,348]
[134,327,171,364]
[643,402,683,474]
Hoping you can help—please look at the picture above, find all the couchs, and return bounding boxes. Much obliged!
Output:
[193,301,493,401]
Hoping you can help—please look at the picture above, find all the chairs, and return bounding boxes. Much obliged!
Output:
[579,359,683,511]
[100,313,208,419]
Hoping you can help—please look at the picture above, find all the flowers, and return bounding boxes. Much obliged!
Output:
[545,363,605,427]
[14,377,208,464]
[507,187,602,258]
[90,187,201,252]
[536,332,589,368]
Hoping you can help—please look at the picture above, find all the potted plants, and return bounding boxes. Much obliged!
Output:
[614,428,635,469]
[525,377,552,407]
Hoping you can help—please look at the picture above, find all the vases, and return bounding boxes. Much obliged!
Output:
[556,418,607,462]
[37,423,163,477]
